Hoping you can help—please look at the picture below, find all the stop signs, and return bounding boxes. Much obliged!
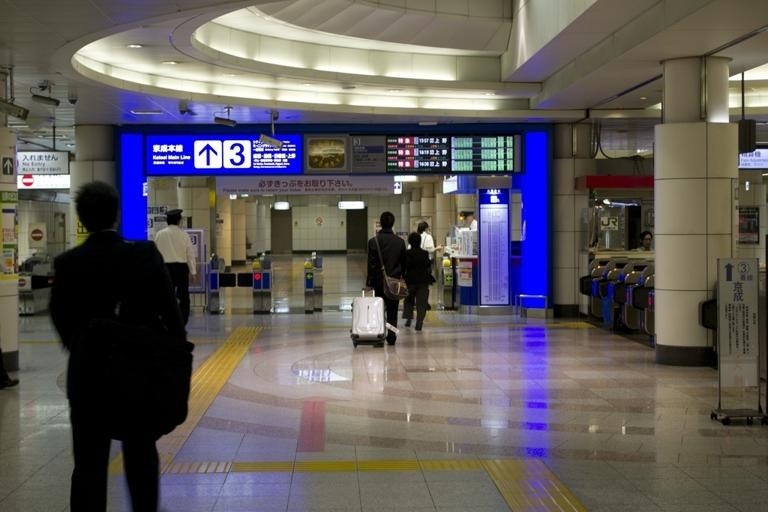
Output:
[22,174,34,186]
[18,278,25,287]
[30,227,43,241]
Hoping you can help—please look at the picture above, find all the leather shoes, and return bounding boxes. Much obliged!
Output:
[405,319,410,326]
[415,320,422,330]
[386,334,396,345]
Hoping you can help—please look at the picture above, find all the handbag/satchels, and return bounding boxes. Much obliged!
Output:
[383,276,409,300]
[423,251,435,284]
[67,310,192,441]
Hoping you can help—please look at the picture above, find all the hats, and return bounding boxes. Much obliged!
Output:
[167,210,182,217]
[459,211,473,216]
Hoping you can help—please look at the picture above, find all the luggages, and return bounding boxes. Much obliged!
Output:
[350,287,388,347]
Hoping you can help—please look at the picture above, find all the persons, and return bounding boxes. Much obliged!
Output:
[402,232,436,331]
[408,221,443,310]
[154,209,197,334]
[48,181,193,512]
[366,211,407,348]
[636,231,654,251]
[451,211,477,251]
